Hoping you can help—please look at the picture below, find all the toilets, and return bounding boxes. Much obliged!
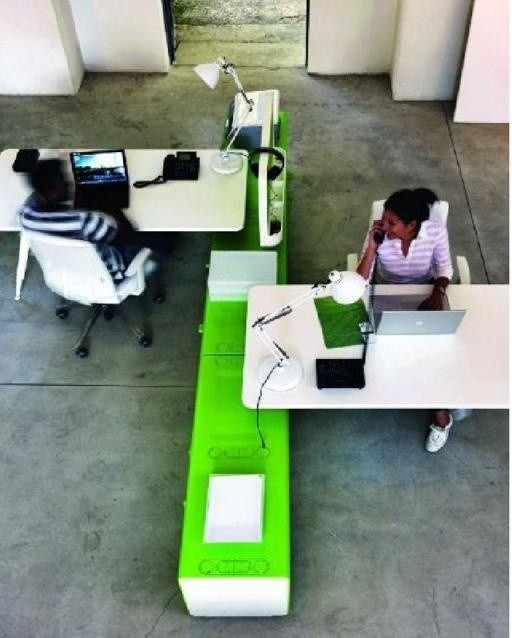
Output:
[163,152,200,181]
[374,228,385,244]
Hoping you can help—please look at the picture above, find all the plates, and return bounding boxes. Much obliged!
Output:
[177,111,289,617]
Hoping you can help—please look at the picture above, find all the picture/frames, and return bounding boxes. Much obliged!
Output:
[0,147,250,258]
[242,284,510,410]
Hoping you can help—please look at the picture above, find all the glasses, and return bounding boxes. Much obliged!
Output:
[192,54,255,176]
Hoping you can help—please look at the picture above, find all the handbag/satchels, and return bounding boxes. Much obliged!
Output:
[434,285,446,295]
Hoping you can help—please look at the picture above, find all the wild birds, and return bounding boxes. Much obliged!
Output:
[69,148,130,212]
[368,292,467,336]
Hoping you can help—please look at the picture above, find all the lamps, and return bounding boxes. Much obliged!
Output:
[344,199,473,286]
[22,231,160,357]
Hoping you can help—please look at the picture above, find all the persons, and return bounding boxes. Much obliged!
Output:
[19,159,173,304]
[353,186,454,453]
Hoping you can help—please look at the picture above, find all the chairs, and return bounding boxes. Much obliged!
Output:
[424,412,453,453]
